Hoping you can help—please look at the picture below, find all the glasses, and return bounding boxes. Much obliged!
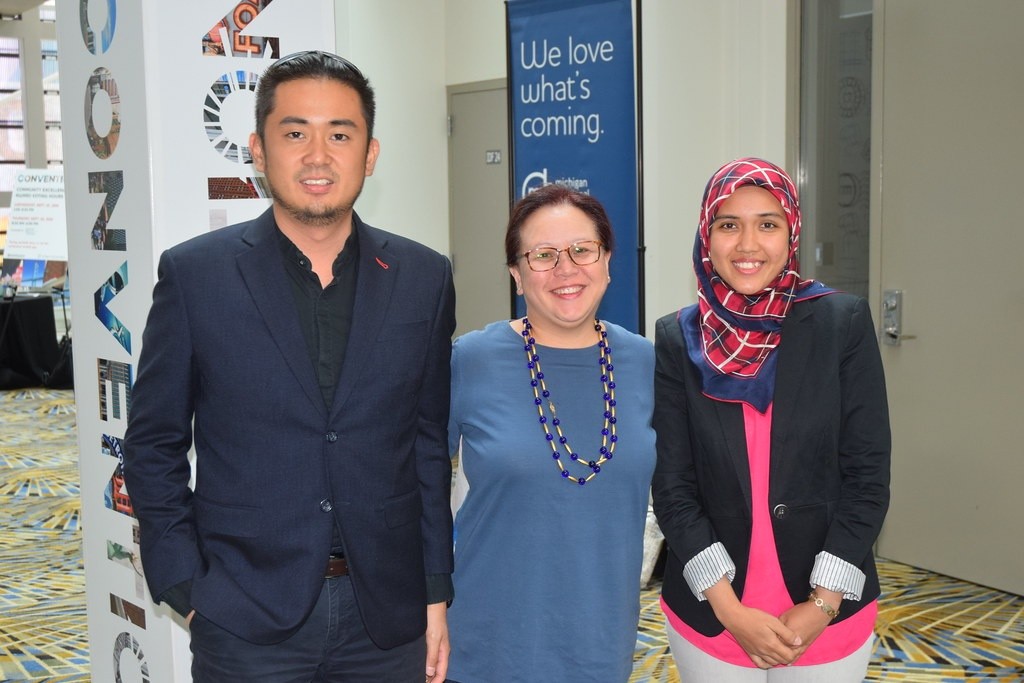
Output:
[270,50,360,74]
[514,241,607,271]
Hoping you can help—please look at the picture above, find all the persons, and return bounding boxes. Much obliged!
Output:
[446,185,658,683]
[652,157,892,683]
[123,51,457,683]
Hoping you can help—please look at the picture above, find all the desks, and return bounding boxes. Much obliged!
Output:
[0,295,58,391]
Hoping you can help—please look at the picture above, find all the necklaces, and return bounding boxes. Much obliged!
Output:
[522,316,618,485]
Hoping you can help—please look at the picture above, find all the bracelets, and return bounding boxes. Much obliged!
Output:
[809,592,839,619]
